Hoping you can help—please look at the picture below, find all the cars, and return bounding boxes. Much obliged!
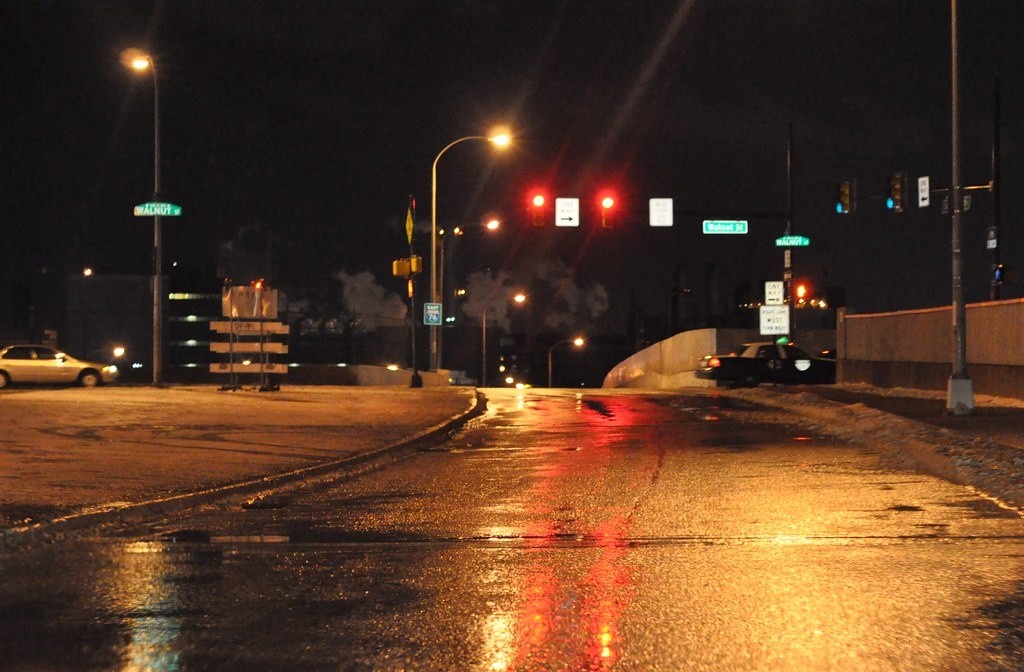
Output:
[694,342,837,387]
[0,343,119,387]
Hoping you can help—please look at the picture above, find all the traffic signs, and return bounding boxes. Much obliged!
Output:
[765,281,784,306]
[918,176,929,206]
[554,198,580,227]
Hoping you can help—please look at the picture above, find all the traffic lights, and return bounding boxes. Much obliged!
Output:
[528,191,546,228]
[793,283,810,300]
[598,193,616,231]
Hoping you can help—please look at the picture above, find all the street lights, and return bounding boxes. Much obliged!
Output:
[548,337,583,387]
[438,220,499,367]
[130,53,164,385]
[427,132,509,374]
